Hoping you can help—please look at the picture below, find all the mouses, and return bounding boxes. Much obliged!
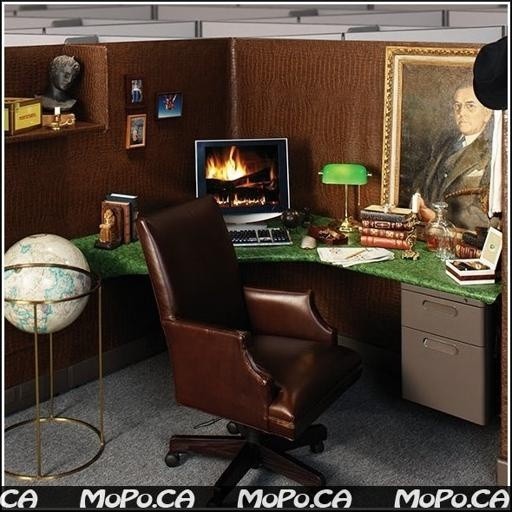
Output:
[299,234,317,251]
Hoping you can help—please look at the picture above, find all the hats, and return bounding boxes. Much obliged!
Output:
[473,36,506,109]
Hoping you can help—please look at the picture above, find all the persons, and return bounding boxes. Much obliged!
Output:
[406,76,502,232]
[32,54,81,113]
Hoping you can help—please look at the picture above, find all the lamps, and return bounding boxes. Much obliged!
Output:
[318,163,374,233]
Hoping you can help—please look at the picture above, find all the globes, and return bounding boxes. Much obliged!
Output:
[3,233,106,481]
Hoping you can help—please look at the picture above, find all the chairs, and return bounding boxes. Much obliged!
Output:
[136,194,362,508]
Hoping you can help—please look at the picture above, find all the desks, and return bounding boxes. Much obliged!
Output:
[69,213,502,430]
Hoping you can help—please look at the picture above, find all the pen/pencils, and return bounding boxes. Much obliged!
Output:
[345,250,364,259]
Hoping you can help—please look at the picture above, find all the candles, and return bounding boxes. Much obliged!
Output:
[54,107,61,117]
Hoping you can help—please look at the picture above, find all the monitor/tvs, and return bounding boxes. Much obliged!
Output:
[194,137,291,226]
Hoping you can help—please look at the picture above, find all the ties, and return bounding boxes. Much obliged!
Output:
[449,136,465,156]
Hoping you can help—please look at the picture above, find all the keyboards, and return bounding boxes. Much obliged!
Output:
[227,225,295,249]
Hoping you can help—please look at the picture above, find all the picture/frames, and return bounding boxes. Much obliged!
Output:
[154,90,184,120]
[379,45,503,246]
[125,114,147,149]
[124,74,148,110]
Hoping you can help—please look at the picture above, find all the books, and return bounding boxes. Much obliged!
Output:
[105,191,140,244]
[100,199,130,245]
[360,204,417,251]
